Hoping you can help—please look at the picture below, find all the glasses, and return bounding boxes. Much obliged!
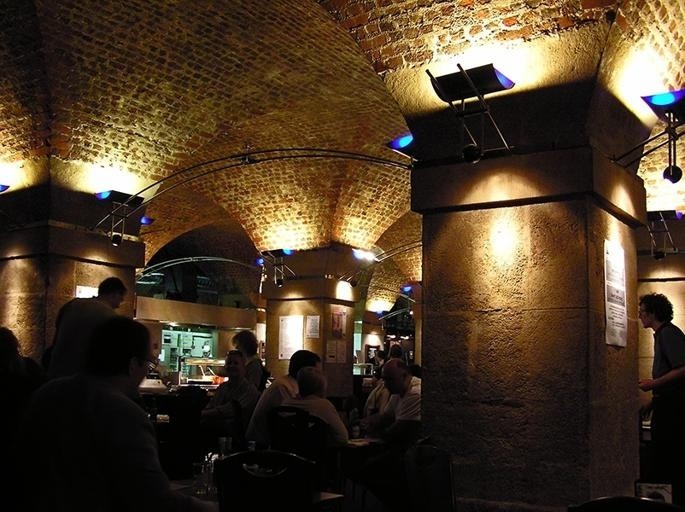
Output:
[639,310,646,316]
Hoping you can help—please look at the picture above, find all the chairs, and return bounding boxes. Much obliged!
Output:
[145,378,462,512]
[564,492,682,512]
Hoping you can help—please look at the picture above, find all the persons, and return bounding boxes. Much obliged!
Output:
[43,275,129,386]
[22,355,43,392]
[39,345,67,382]
[635,291,684,509]
[197,330,423,492]
[0,326,33,480]
[0,315,222,512]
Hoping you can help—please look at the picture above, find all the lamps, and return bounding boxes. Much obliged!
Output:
[423,64,517,162]
[645,211,683,258]
[615,88,685,183]
[256,247,414,317]
